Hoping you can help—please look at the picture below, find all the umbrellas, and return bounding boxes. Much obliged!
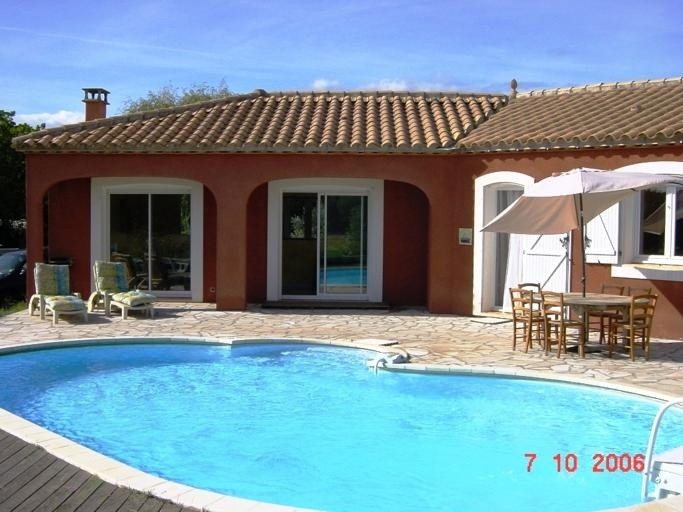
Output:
[479,169,682,355]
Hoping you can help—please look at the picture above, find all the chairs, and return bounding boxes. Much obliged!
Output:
[112,251,152,289]
[88,261,155,320]
[28,261,90,326]
[507,281,656,361]
[145,251,189,290]
